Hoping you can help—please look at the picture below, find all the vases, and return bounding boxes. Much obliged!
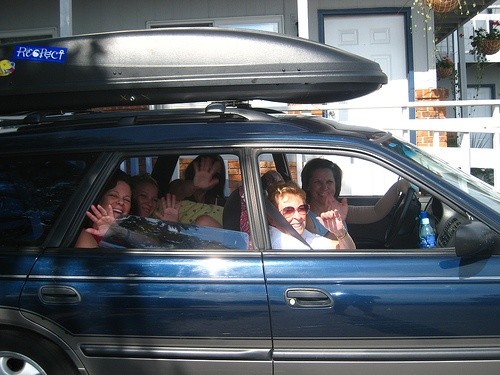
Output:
[482,40,500,55]
[437,68,453,78]
[426,0,458,12]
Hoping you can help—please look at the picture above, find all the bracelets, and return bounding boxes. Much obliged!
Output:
[337,229,348,240]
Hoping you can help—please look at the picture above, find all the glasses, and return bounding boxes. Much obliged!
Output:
[278,204,311,217]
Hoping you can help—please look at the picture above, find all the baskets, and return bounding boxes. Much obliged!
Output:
[436,63,453,77]
[476,40,500,55]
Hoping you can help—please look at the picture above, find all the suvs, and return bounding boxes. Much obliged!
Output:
[0,26,500,375]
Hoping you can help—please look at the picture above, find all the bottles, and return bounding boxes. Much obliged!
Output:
[418,212,437,248]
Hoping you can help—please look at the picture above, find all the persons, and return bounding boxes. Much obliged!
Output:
[267,180,357,250]
[300,159,410,237]
[74,169,134,248]
[165,153,226,229]
[130,176,221,228]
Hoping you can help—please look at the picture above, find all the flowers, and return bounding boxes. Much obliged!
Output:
[410,0,480,54]
[435,55,463,118]
[469,20,500,115]
